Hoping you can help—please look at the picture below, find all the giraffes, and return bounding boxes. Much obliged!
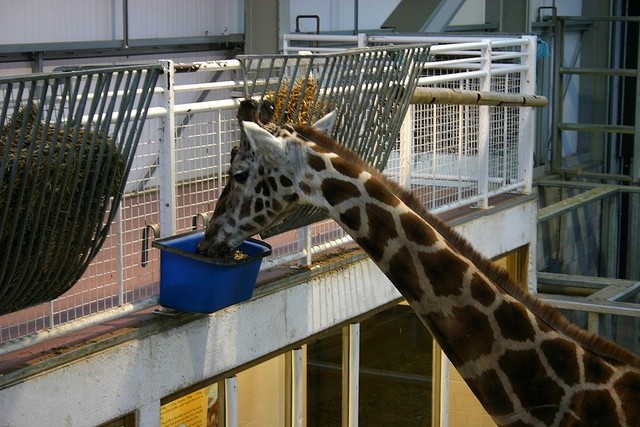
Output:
[196,96,640,427]
